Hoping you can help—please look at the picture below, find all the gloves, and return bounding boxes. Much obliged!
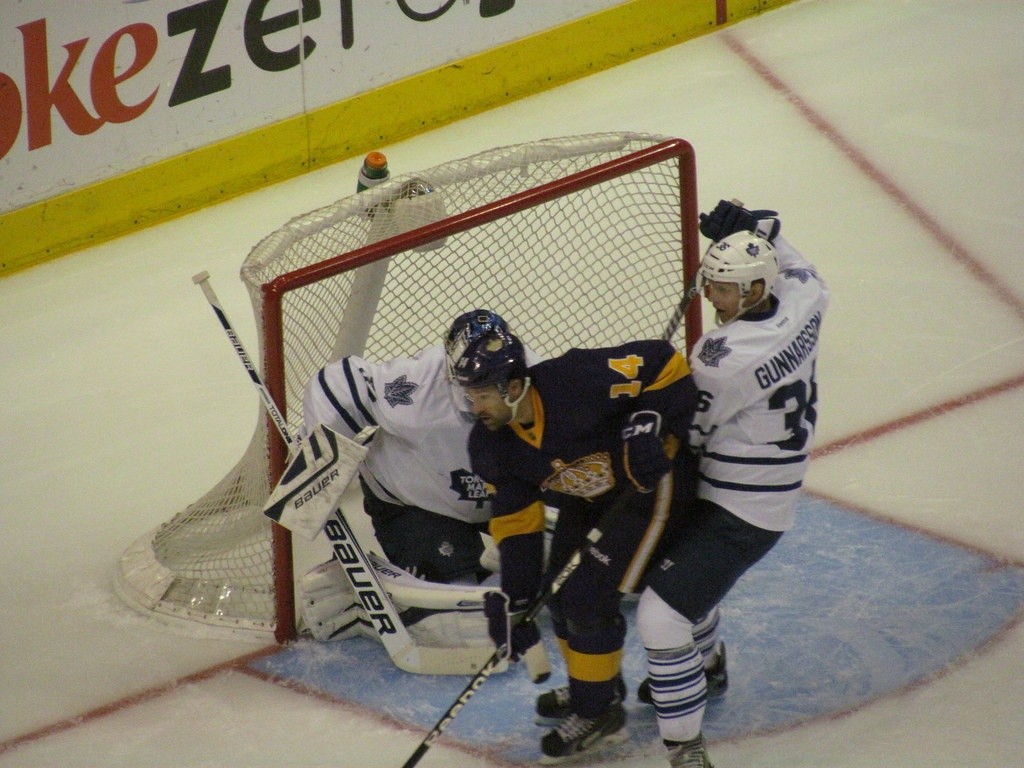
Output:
[627,433,671,489]
[700,200,760,241]
[488,612,541,663]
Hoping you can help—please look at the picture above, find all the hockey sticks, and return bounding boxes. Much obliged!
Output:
[402,492,626,767]
[661,198,744,341]
[191,269,509,676]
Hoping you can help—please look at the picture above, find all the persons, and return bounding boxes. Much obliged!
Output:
[634,201,829,768]
[296,308,559,644]
[445,323,701,765]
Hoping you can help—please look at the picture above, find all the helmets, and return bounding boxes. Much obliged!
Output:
[699,229,779,327]
[454,333,527,395]
[449,310,510,349]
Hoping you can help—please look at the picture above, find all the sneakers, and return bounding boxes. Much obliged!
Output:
[639,642,729,707]
[663,730,712,768]
[539,691,632,767]
[534,678,628,725]
